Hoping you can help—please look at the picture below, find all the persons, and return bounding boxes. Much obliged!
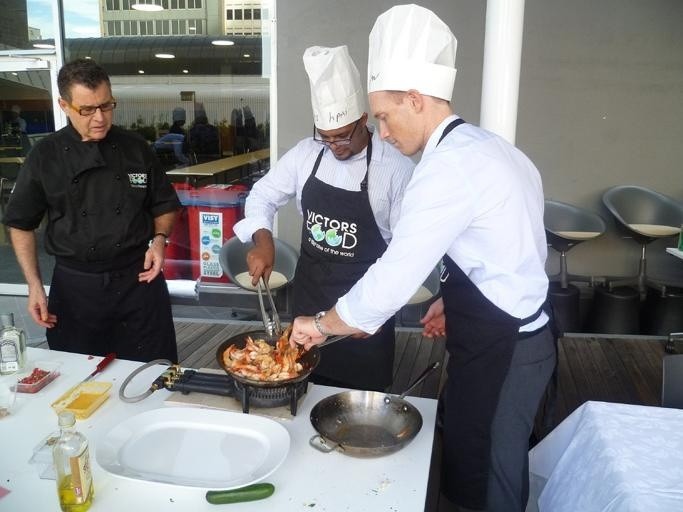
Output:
[290,4,559,511]
[154,101,265,169]
[0,60,183,364]
[7,101,26,132]
[232,46,413,386]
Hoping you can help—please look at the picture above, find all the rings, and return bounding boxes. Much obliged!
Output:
[160,268,164,272]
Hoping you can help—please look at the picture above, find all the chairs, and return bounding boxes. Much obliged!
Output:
[221,233,300,322]
[602,184,682,298]
[396,265,441,328]
[541,199,608,294]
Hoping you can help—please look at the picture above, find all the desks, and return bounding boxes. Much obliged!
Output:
[548,399,682,511]
[0,344,438,512]
[164,148,270,187]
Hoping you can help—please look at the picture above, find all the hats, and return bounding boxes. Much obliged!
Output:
[366,2,459,104]
[301,44,366,132]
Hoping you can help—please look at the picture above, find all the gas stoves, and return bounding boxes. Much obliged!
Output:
[167,368,307,416]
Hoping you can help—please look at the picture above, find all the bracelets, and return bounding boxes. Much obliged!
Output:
[152,233,172,245]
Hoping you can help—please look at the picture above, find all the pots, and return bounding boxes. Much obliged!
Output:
[308,359,444,460]
[215,325,357,387]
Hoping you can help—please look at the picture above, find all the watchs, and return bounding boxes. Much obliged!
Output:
[315,310,330,338]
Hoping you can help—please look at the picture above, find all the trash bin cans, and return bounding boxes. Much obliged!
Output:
[152,182,251,283]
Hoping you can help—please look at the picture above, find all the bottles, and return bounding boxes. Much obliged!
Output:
[0,312,27,374]
[52,410,95,512]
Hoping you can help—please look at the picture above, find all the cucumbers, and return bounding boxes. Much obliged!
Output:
[206,483,275,504]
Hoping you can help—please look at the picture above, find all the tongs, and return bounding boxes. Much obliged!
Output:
[256,275,282,338]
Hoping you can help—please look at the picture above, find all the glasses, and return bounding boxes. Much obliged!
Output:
[68,96,118,117]
[313,120,358,147]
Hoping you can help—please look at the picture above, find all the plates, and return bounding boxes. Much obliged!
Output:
[99,402,290,491]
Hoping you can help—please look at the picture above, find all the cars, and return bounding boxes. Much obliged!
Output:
[0,145,46,250]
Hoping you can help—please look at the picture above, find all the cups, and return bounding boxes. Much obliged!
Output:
[0,376,17,417]
